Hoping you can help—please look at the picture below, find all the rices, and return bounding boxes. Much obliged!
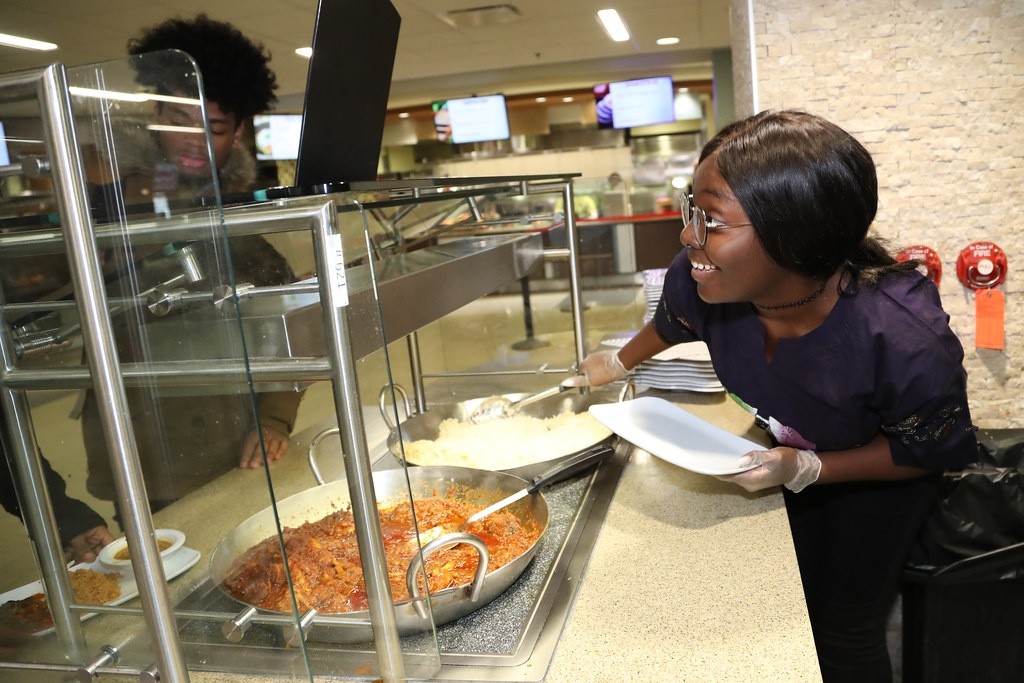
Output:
[397,410,612,470]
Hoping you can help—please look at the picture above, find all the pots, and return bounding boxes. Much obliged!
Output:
[207,426,549,643]
[378,382,626,484]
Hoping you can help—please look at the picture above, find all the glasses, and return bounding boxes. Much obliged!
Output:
[680,192,755,246]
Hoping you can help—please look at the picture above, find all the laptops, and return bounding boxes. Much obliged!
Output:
[93,0,402,222]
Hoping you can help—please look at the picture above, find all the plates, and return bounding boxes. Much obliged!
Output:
[0,547,202,644]
[599,336,725,393]
[99,528,186,567]
[643,267,670,326]
[588,396,770,477]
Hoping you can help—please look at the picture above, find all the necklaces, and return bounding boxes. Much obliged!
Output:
[756,282,826,311]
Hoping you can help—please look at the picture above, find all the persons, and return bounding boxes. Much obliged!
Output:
[561,108,972,683]
[76,15,310,534]
[0,396,113,564]
